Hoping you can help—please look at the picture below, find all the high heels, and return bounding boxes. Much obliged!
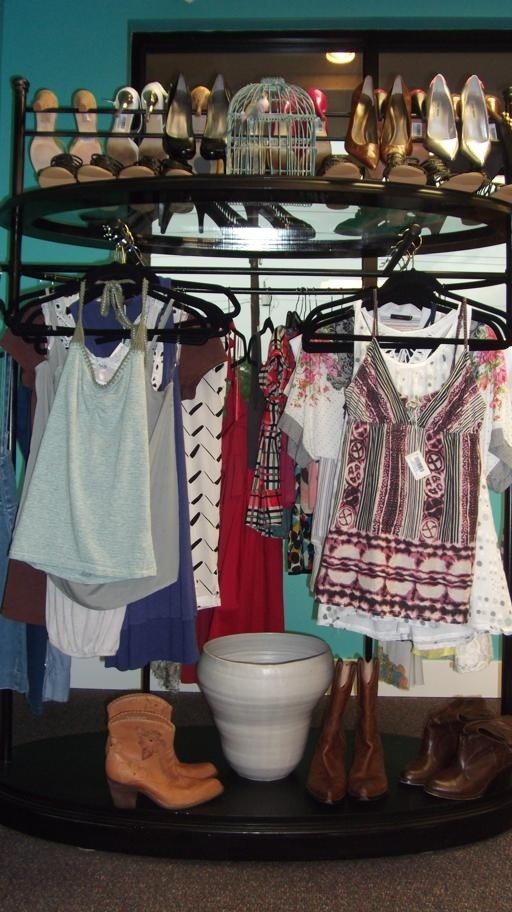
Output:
[323,74,512,202]
[79,199,315,239]
[329,204,449,234]
[31,72,233,187]
[241,86,332,177]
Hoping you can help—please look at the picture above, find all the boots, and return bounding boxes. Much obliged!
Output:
[105,693,224,810]
[306,657,354,806]
[399,699,511,800]
[350,656,390,804]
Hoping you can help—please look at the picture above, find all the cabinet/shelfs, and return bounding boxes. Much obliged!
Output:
[0,69,511,870]
